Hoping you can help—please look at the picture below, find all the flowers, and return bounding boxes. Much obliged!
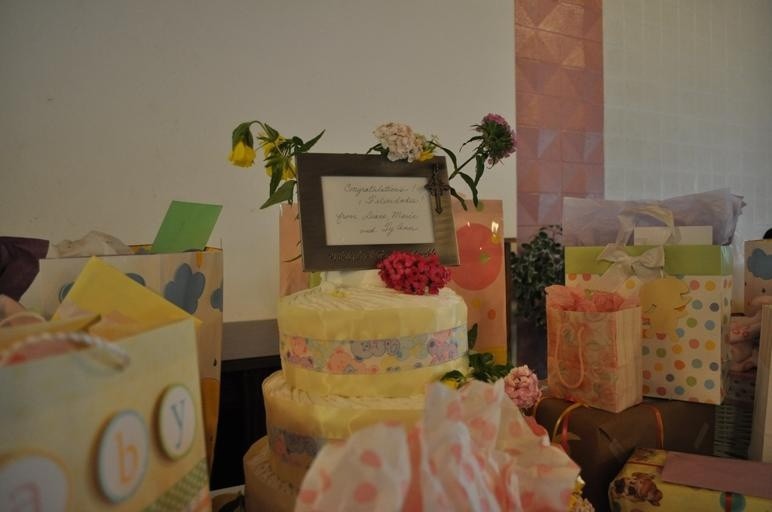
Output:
[376,247,451,295]
[365,112,520,210]
[229,119,326,210]
[438,319,543,413]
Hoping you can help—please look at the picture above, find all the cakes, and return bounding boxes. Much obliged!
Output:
[243,253,513,512]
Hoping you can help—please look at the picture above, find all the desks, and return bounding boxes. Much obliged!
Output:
[530,380,715,512]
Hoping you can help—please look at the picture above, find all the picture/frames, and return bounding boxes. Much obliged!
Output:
[296,153,460,272]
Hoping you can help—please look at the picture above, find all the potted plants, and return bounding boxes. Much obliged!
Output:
[508,223,565,381]
